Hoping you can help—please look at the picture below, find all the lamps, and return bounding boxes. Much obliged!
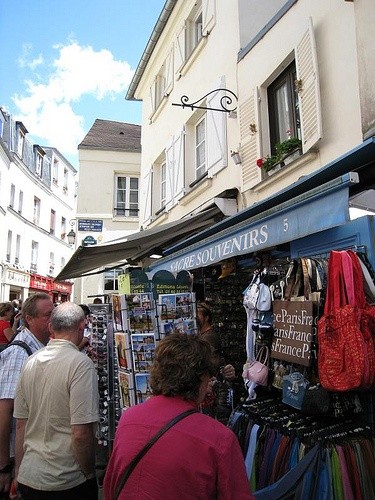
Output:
[67,219,77,245]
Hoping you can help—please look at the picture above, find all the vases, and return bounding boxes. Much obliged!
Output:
[281,147,302,165]
[266,162,284,176]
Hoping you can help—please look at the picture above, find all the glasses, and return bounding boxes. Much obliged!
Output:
[88,316,108,441]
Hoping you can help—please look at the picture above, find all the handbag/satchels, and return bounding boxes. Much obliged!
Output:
[271,360,286,389]
[248,346,270,387]
[213,366,233,418]
[246,265,271,311]
[281,362,310,410]
[317,249,375,393]
[271,257,329,367]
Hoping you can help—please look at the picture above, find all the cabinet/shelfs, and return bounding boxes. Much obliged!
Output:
[87,292,198,486]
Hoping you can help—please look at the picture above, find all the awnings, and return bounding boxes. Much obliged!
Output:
[52,207,220,283]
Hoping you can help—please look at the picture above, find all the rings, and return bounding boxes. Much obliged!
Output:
[231,371,233,374]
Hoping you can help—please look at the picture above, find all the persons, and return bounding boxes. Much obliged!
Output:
[102,302,256,500]
[0,293,102,500]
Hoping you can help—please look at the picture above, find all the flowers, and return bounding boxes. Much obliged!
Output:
[274,129,302,154]
[257,154,279,170]
